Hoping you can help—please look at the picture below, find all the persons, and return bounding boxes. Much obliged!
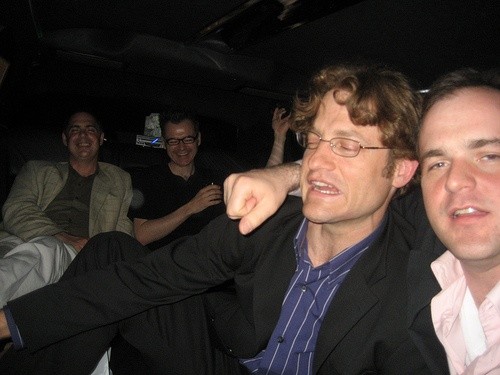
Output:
[0,64,446,375]
[126,107,226,253]
[225,73,500,375]
[0,111,136,311]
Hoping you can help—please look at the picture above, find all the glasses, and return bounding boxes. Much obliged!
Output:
[164,133,199,146]
[296,129,417,157]
